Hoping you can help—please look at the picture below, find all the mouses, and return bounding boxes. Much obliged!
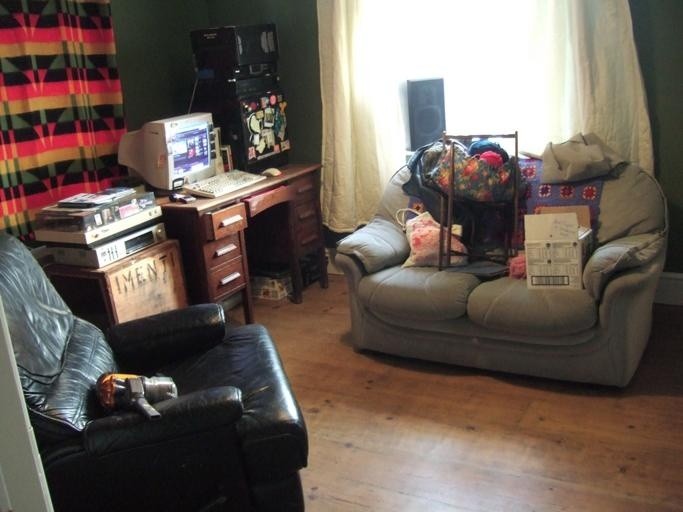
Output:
[262,167,282,177]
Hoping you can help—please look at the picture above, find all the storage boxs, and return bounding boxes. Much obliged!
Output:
[519,206,595,290]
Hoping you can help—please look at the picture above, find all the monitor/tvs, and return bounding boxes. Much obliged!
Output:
[116,112,218,198]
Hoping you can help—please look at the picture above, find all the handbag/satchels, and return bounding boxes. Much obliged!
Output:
[407,139,528,202]
[396,208,468,268]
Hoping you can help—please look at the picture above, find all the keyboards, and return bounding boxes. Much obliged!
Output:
[182,169,267,199]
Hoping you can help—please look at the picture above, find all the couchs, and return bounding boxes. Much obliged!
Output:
[332,144,667,389]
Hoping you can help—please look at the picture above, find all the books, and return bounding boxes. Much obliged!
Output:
[33,185,167,269]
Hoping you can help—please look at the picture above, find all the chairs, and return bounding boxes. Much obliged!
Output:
[435,129,523,275]
[2,229,310,512]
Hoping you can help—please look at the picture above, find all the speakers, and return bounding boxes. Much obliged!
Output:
[407,77,446,151]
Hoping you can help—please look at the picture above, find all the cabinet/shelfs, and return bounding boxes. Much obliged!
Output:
[36,240,189,328]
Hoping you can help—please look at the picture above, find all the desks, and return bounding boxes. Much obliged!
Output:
[143,160,324,327]
